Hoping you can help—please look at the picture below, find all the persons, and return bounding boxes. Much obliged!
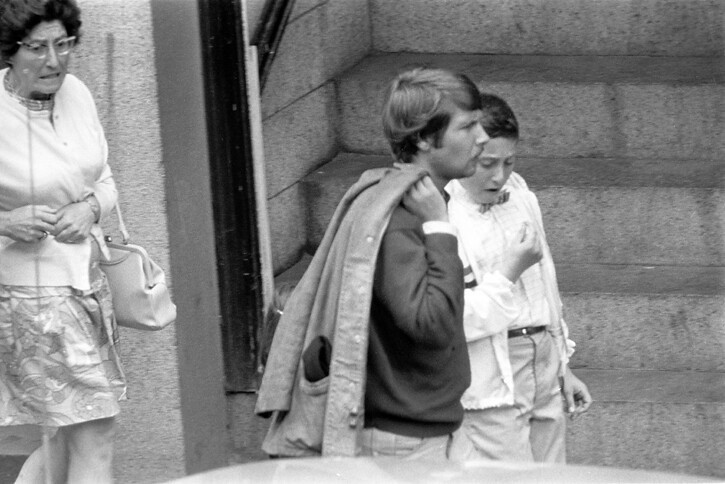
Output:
[446,93,593,465]
[0,0,128,484]
[358,66,490,460]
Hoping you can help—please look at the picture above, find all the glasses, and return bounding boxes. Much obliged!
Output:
[16,36,75,58]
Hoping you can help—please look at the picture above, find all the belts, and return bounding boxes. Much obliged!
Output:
[508,325,545,337]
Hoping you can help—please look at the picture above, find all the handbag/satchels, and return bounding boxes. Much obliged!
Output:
[83,184,176,330]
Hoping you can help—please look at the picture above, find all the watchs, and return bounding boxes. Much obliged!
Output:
[85,198,100,222]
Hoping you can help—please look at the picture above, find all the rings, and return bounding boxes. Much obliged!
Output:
[40,232,47,240]
